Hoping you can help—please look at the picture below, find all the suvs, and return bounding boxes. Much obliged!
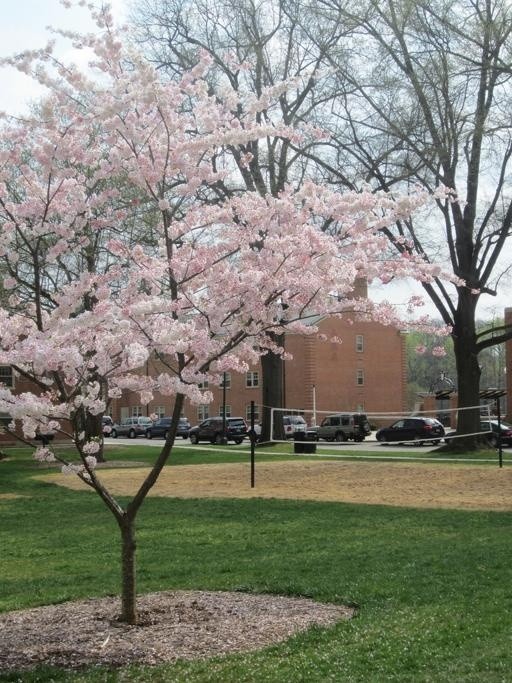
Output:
[315,412,373,443]
[102,413,308,446]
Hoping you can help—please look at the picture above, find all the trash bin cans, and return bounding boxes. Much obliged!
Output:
[306,431,317,453]
[293,432,305,452]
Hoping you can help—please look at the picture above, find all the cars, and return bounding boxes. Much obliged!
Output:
[374,416,447,447]
[307,424,321,437]
[443,418,512,449]
